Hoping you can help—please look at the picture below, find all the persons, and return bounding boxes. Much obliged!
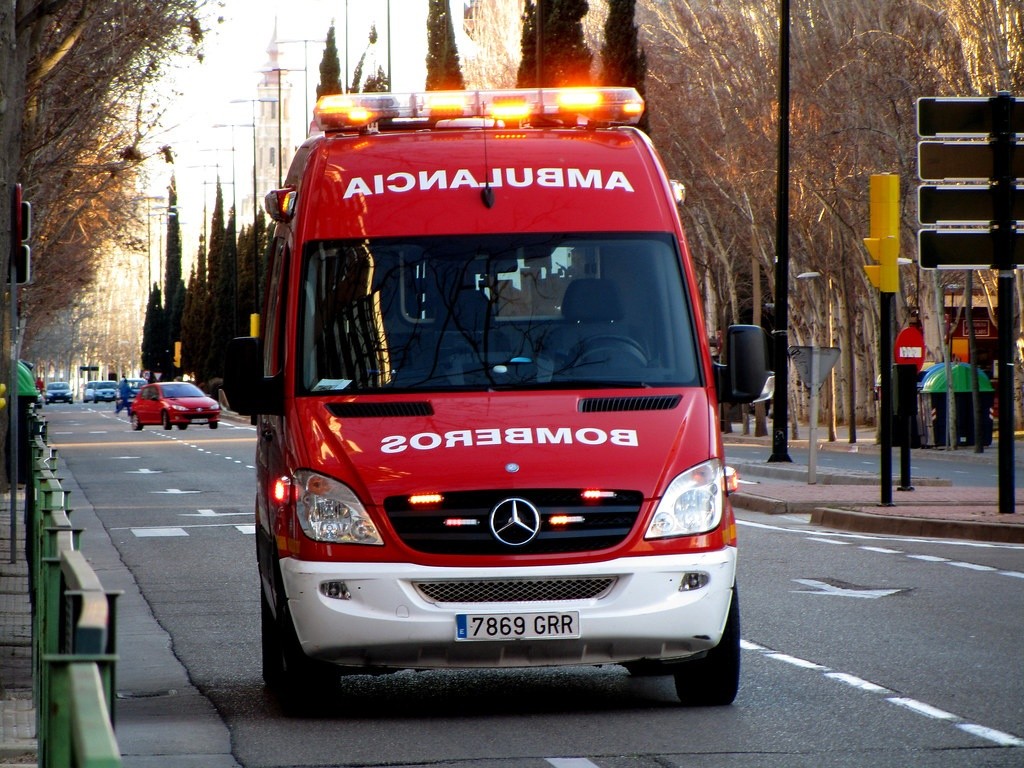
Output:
[36,377,44,395]
[116,380,137,416]
[148,372,156,383]
[119,375,126,384]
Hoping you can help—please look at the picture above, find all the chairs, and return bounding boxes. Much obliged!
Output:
[536,279,652,385]
[396,289,535,385]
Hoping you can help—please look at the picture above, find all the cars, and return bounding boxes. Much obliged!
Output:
[116,378,148,410]
[45,382,73,405]
[84,381,99,403]
[93,380,120,403]
[130,381,220,431]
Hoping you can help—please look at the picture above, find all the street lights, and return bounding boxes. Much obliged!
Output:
[176,34,310,429]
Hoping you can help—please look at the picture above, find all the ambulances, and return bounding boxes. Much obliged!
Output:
[224,83,768,705]
